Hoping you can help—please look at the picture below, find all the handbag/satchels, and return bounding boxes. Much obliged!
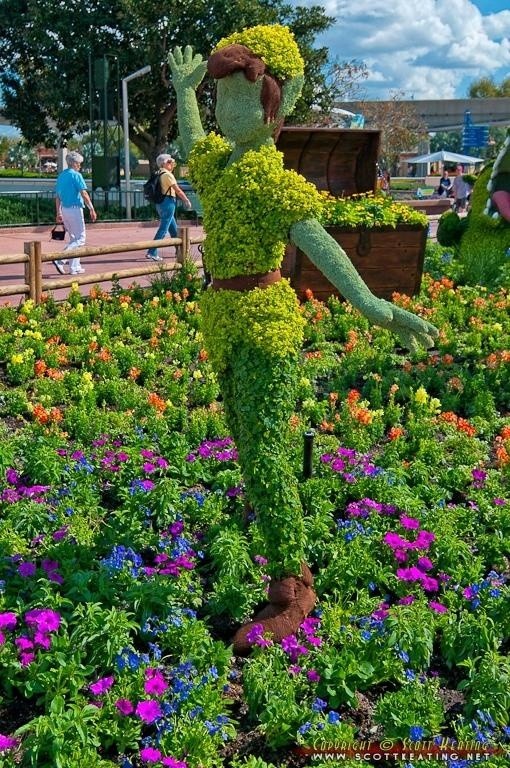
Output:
[52,230,65,240]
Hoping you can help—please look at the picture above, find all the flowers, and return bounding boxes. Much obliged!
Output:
[301,183,426,230]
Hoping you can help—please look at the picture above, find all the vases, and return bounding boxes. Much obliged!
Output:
[287,222,429,299]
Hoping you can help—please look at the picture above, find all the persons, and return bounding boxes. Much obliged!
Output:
[448,165,473,213]
[380,169,390,192]
[145,153,191,261]
[438,170,453,198]
[52,151,97,275]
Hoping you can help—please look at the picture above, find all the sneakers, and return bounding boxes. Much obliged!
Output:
[53,260,85,274]
[146,254,163,261]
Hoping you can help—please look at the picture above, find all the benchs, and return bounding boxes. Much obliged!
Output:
[175,193,204,227]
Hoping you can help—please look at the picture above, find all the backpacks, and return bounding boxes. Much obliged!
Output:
[143,170,171,204]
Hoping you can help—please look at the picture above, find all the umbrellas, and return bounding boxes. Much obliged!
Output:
[400,149,485,177]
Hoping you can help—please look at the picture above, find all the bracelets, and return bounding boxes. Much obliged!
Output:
[87,203,93,209]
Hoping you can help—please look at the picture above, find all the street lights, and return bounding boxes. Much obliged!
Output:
[116,63,153,220]
